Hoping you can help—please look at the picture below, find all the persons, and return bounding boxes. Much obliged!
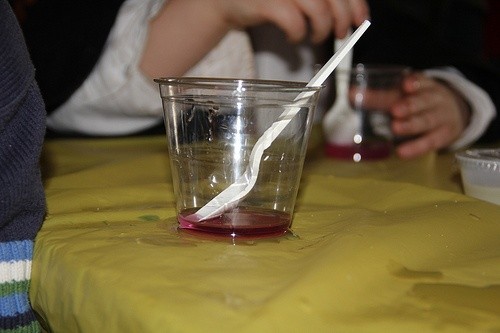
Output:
[0,0,51,333]
[8,0,500,159]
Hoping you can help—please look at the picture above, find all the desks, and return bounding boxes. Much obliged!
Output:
[28,124,500,333]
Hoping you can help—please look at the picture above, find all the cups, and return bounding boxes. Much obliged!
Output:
[313,62,409,160]
[151,77,326,244]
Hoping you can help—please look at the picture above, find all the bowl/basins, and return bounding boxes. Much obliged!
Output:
[455,147,500,206]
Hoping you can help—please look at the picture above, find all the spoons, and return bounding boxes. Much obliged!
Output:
[322,28,363,148]
[188,20,371,222]
